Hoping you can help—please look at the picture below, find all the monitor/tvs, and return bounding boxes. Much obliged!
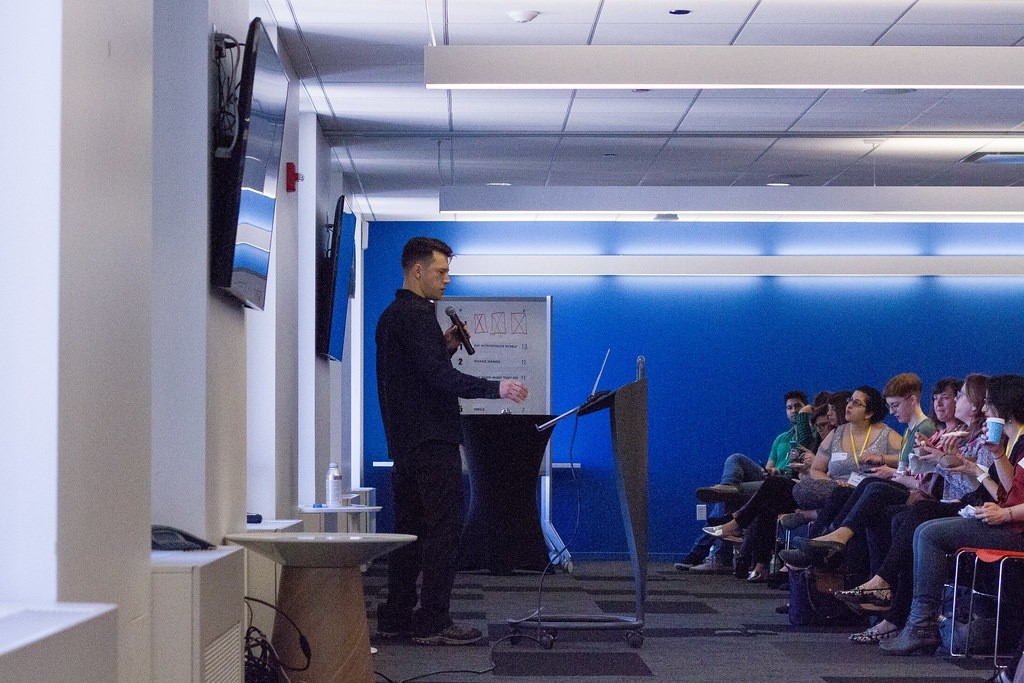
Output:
[211,17,291,312]
[319,194,355,362]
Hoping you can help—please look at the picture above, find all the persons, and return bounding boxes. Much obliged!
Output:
[674,373,1024,656]
[374,238,528,644]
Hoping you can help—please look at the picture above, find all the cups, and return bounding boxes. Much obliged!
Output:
[985,418,1005,444]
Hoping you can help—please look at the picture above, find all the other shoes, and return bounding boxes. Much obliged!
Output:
[689,558,733,574]
[695,483,740,503]
[780,512,807,530]
[702,524,744,543]
[769,564,792,582]
[746,568,767,581]
[833,584,893,612]
[778,547,811,572]
[676,561,695,570]
[775,604,792,614]
[848,625,904,643]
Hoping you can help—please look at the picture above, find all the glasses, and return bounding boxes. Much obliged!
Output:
[814,422,828,429]
[886,395,912,411]
[957,391,967,400]
[847,397,867,408]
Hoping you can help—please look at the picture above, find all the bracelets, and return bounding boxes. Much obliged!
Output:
[880,453,885,465]
[1008,508,1014,522]
[992,453,1006,460]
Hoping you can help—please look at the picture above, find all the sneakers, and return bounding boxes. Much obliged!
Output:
[376,626,414,638]
[411,623,483,646]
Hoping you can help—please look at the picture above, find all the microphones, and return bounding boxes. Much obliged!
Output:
[445,306,476,356]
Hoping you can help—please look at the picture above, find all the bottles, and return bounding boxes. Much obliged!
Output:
[733,547,739,568]
[770,555,780,574]
[326,463,342,508]
[897,462,906,478]
[710,543,716,559]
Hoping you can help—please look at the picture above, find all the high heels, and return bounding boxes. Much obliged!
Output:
[792,536,846,569]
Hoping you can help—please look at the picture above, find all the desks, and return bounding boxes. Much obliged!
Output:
[455,413,559,574]
[225,532,419,683]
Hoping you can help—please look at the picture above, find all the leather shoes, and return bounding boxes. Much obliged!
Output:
[876,622,942,656]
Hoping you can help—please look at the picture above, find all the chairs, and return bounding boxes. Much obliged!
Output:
[771,509,1024,670]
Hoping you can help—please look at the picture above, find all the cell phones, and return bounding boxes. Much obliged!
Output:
[864,469,877,473]
[790,441,804,455]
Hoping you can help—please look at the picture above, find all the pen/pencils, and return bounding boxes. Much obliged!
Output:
[758,460,768,473]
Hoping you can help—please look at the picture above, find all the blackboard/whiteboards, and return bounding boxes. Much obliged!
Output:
[433,295,552,417]
